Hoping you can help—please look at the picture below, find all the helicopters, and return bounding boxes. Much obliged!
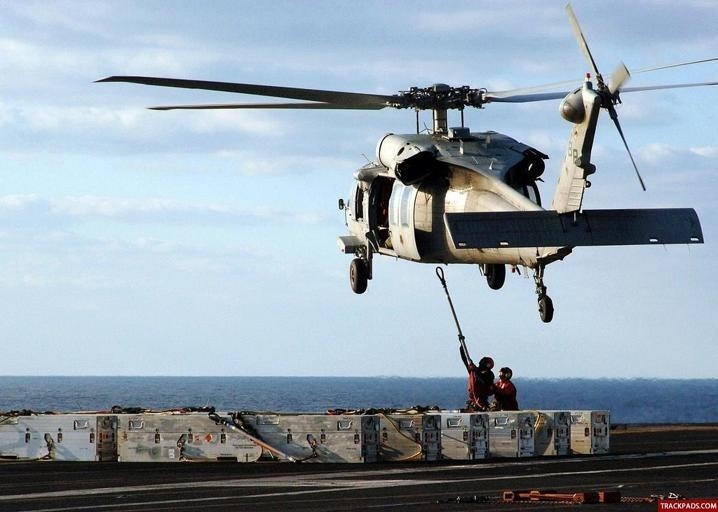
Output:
[93,2,718,323]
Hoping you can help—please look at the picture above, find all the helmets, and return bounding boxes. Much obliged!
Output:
[479,356,494,368]
[500,367,513,379]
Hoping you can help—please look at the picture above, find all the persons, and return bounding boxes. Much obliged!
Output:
[488,366,519,411]
[457,334,496,411]
